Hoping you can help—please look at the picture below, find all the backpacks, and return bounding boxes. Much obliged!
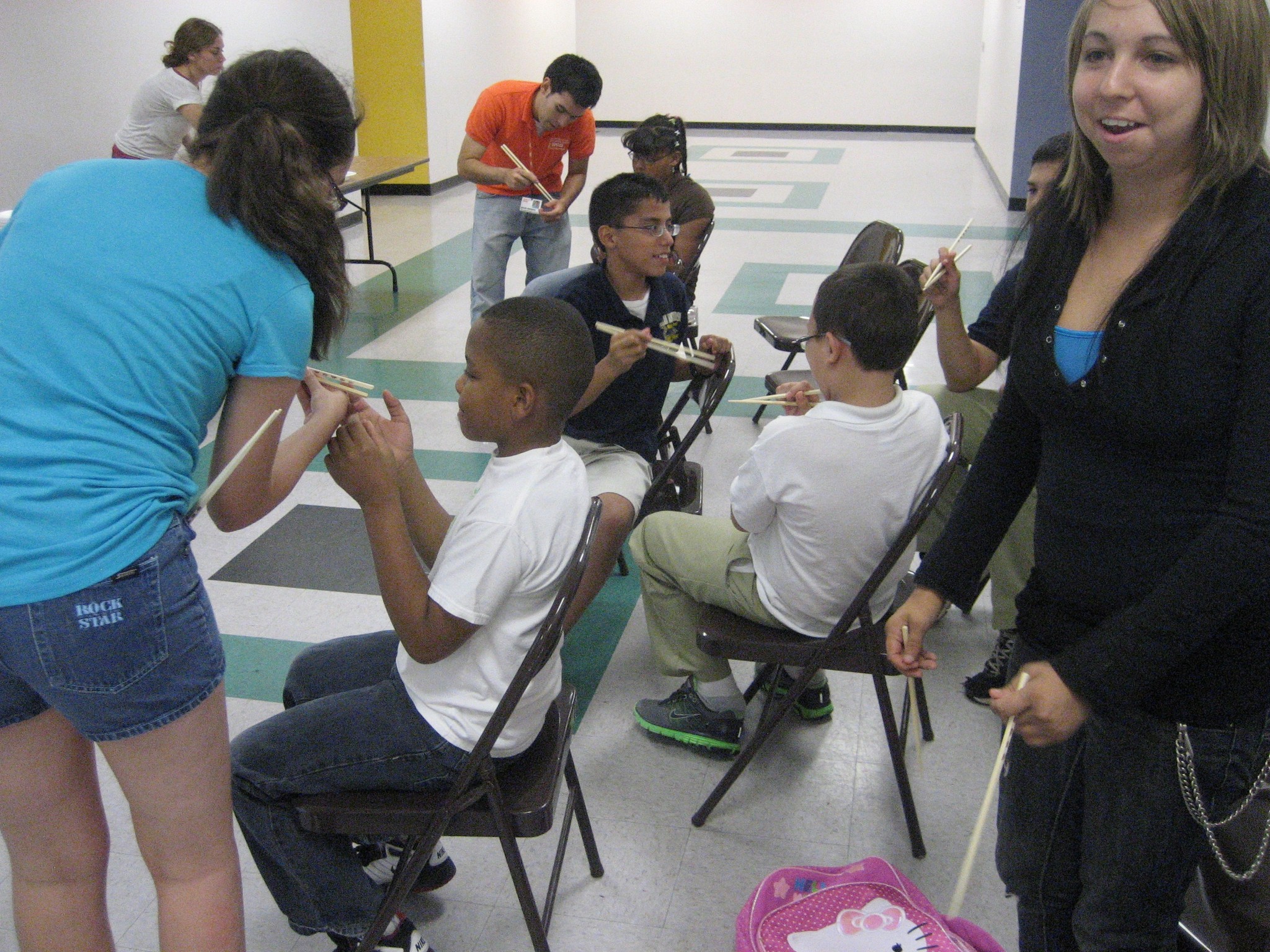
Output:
[734,856,1004,952]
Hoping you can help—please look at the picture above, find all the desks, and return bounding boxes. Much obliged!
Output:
[324,157,433,292]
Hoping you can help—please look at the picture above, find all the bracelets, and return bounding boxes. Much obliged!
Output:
[689,363,700,378]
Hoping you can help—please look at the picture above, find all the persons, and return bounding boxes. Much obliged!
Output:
[0,48,366,951]
[628,260,950,758]
[457,54,603,327]
[907,131,1074,707]
[620,112,715,308]
[554,172,732,637]
[883,0,1269,952]
[111,17,226,160]
[229,296,597,952]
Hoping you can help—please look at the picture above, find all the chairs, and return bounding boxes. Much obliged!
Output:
[296,490,634,952]
[750,221,944,423]
[694,414,963,856]
[679,222,716,351]
[613,347,740,576]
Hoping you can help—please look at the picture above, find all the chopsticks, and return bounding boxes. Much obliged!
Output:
[900,625,1030,925]
[500,143,554,203]
[728,389,822,408]
[185,408,283,525]
[307,367,375,398]
[922,217,973,292]
[595,321,716,370]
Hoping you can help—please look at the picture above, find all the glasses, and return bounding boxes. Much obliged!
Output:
[799,333,852,350]
[628,150,675,162]
[313,151,348,213]
[610,224,680,237]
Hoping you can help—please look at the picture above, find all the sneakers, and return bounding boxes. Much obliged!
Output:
[633,675,744,756]
[755,661,834,718]
[357,834,456,893]
[341,914,434,952]
[962,628,1024,703]
